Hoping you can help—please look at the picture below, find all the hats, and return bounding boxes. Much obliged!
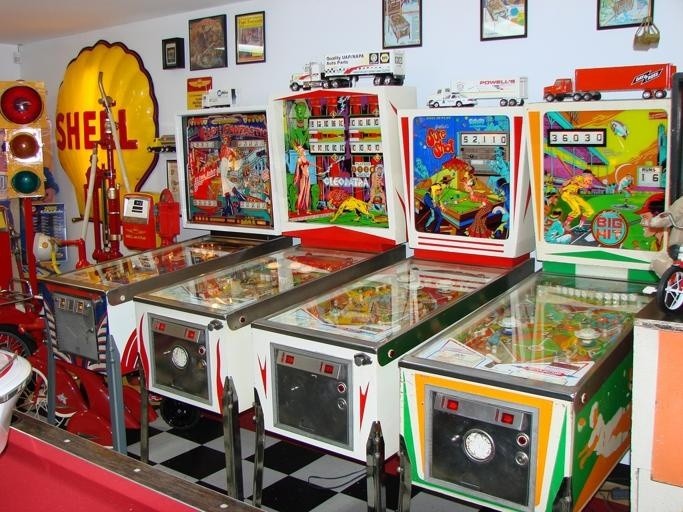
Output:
[634,194,664,215]
[37,167,60,200]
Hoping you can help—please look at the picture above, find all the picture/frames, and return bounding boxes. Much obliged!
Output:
[381,0,422,50]
[596,0,654,31]
[160,12,266,71]
[479,0,527,42]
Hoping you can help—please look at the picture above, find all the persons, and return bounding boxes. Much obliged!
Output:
[423,147,510,241]
[618,174,635,208]
[366,167,386,214]
[543,170,594,252]
[632,193,664,252]
[293,145,324,216]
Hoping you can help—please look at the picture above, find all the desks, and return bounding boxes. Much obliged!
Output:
[0,407,265,512]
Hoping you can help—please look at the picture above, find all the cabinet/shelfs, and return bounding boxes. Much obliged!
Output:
[630,293,682,512]
